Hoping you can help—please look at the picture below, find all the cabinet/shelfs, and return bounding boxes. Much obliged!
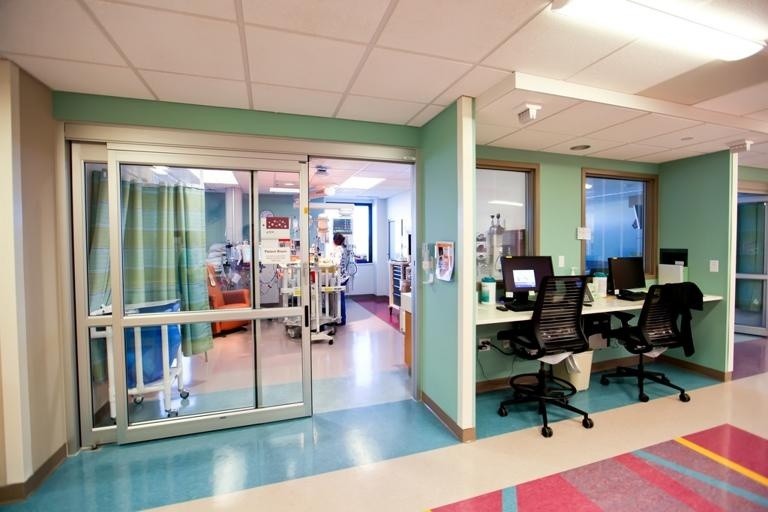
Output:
[387,259,409,317]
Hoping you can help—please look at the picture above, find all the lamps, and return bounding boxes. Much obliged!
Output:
[513,102,541,125]
[550,0,768,62]
[726,139,755,154]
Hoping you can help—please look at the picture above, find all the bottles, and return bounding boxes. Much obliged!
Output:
[593,272,607,298]
[481,277,496,304]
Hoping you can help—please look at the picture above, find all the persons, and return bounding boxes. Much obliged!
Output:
[332,231,351,327]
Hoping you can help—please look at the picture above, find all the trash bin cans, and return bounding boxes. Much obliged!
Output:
[551,350,594,391]
[89,298,188,426]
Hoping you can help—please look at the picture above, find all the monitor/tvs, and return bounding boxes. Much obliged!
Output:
[500,256,557,302]
[608,257,646,295]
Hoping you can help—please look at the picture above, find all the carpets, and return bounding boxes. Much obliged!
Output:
[430,422,768,512]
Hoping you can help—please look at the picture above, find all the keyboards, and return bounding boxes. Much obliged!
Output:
[617,294,661,302]
[504,301,535,312]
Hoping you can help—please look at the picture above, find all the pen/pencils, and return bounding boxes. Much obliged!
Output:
[583,304,592,307]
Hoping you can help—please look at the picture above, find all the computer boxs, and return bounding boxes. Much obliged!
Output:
[658,264,689,285]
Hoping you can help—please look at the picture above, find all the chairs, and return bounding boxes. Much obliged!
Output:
[599,284,692,403]
[206,264,252,335]
[496,274,594,438]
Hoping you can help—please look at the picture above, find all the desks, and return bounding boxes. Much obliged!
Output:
[404,310,413,376]
[475,290,723,325]
[399,292,413,335]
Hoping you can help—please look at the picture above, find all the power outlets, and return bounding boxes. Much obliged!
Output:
[479,338,491,351]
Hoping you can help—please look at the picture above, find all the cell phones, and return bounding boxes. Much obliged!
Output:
[496,306,508,311]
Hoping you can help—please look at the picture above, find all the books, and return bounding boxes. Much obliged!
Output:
[656,263,683,285]
[683,266,689,283]
